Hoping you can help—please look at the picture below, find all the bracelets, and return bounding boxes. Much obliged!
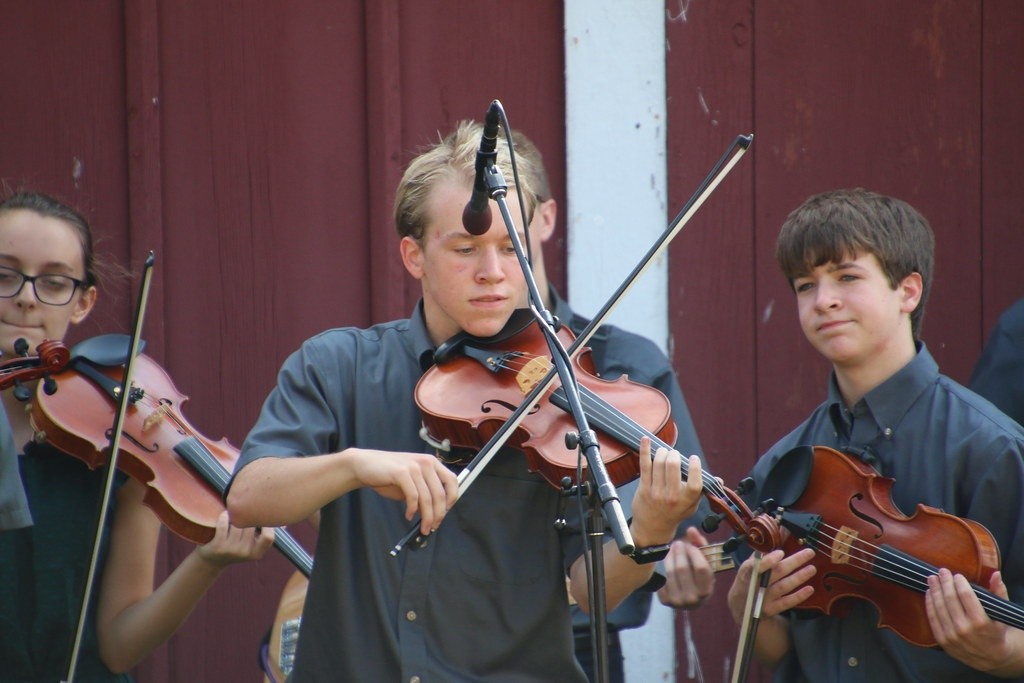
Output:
[627,516,672,564]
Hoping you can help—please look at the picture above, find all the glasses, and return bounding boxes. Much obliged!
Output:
[0,266,86,306]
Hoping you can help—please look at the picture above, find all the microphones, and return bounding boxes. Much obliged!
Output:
[462,100,501,235]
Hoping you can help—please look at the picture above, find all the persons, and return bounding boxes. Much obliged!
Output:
[728,187,1024,683]
[0,193,286,683]
[225,123,724,683]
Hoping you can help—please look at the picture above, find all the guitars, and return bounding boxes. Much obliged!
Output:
[261,536,738,683]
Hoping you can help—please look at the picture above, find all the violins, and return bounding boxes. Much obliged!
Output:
[411,306,789,556]
[0,335,70,405]
[753,440,1023,654]
[28,330,315,582]
